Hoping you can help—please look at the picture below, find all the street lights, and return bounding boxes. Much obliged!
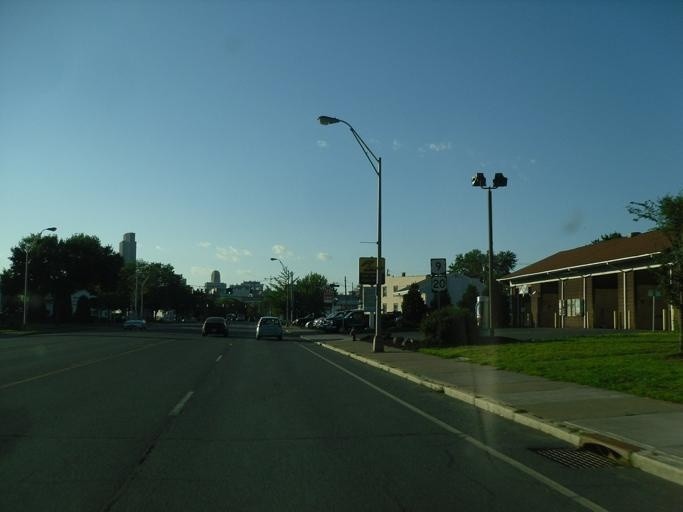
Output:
[270,257,290,327]
[317,115,385,353]
[469,171,509,343]
[23,226,57,325]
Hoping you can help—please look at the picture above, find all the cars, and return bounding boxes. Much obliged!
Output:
[255,317,283,340]
[293,309,402,333]
[201,316,229,337]
[123,318,148,330]
